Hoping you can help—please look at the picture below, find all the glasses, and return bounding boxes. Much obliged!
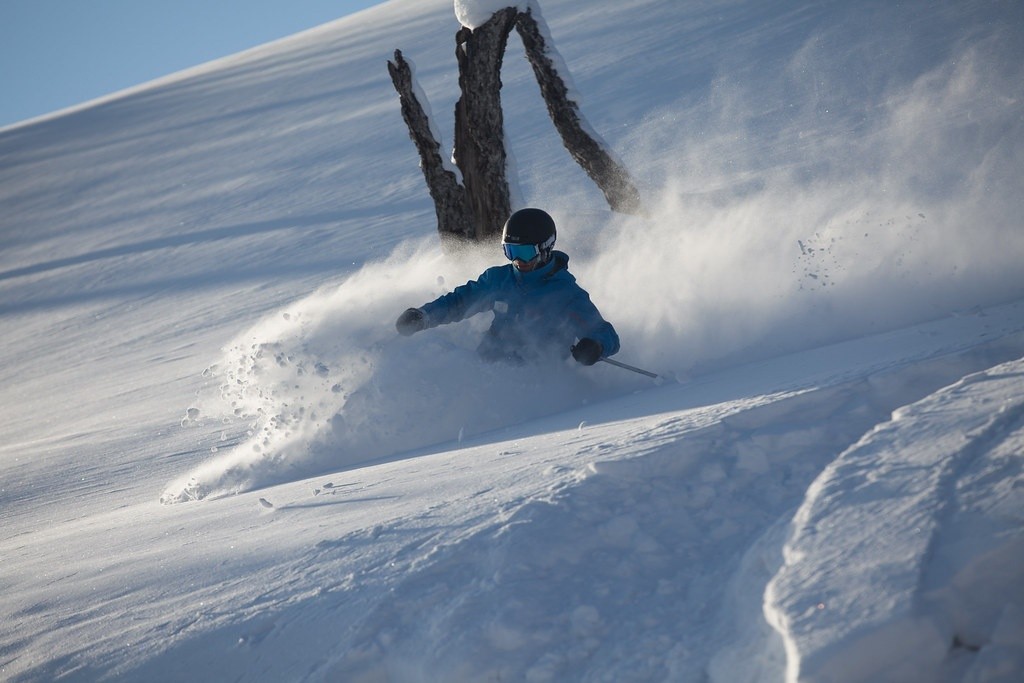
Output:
[502,234,554,262]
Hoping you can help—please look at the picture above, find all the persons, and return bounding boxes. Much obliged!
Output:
[395,208,620,366]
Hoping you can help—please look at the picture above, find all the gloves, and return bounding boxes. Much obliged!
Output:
[396,308,425,337]
[573,337,602,367]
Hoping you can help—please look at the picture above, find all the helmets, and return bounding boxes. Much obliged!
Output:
[503,208,556,251]
